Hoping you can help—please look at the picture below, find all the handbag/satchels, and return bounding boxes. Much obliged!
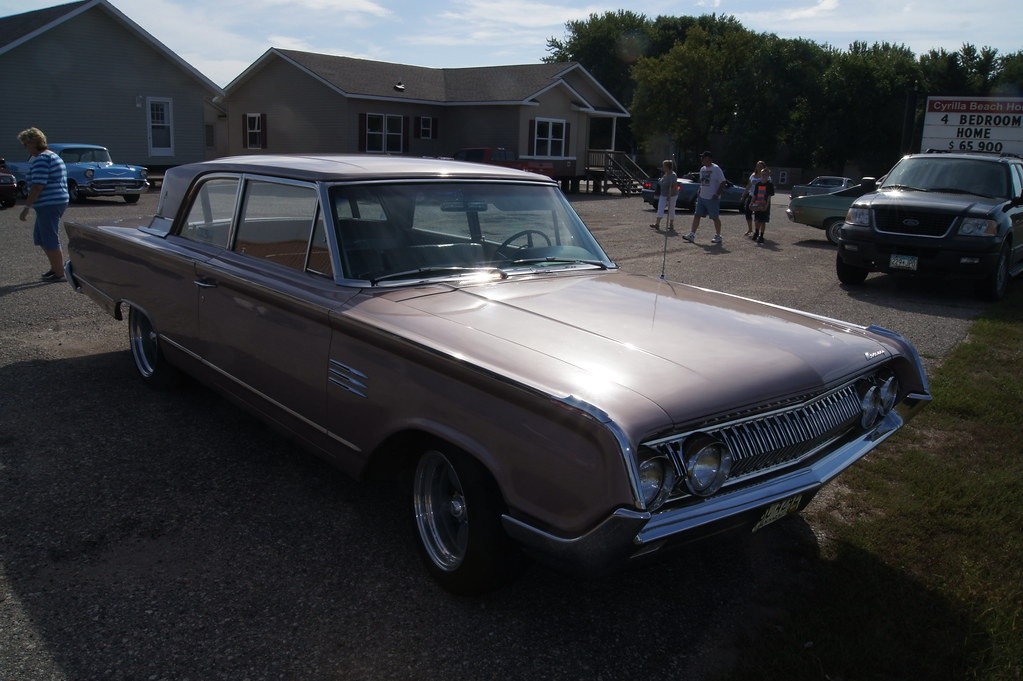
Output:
[653,183,661,199]
[749,202,767,211]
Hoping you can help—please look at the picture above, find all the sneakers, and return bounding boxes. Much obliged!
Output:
[682,233,695,242]
[711,234,722,242]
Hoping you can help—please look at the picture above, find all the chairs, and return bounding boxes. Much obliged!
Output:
[405,243,484,270]
[344,246,385,281]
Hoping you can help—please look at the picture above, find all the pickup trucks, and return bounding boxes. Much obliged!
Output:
[454,147,555,178]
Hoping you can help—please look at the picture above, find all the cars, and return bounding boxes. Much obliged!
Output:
[791,175,856,197]
[6,143,151,204]
[0,158,18,208]
[641,171,752,214]
[785,183,882,247]
[61,152,937,598]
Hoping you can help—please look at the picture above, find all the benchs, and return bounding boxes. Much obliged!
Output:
[138,165,183,189]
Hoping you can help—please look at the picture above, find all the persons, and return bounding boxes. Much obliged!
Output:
[682,150,726,243]
[650,160,679,231]
[741,160,775,243]
[17,126,70,282]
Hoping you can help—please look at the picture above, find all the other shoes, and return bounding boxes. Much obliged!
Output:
[41,270,54,276]
[753,232,757,241]
[758,235,764,243]
[42,275,67,282]
[745,230,753,235]
[668,226,673,231]
[650,224,659,230]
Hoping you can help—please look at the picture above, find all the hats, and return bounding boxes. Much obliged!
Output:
[699,151,712,158]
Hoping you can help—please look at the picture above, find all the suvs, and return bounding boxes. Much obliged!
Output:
[834,149,1023,303]
